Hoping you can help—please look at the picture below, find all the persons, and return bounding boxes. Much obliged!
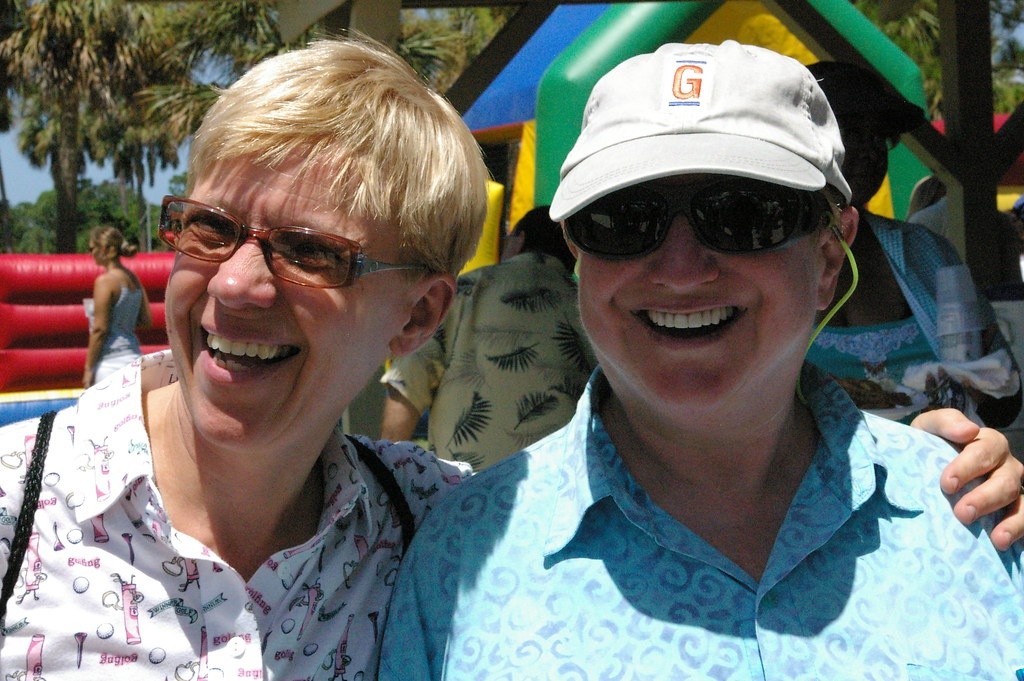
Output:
[82,224,154,389]
[898,173,1024,303]
[0,34,1024,680]
[376,204,597,476]
[803,61,1022,439]
[371,41,1024,680]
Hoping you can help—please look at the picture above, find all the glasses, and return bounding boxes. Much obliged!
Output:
[838,126,901,152]
[158,195,435,288]
[565,186,834,260]
[89,245,95,253]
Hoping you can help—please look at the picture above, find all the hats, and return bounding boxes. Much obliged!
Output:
[805,61,926,135]
[549,39,852,223]
